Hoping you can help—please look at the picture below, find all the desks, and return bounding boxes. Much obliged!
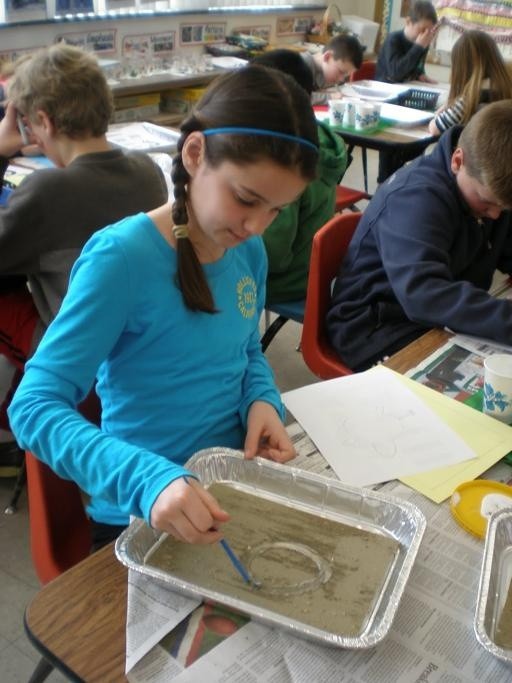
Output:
[2,35,512,683]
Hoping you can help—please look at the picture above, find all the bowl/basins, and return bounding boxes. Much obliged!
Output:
[203,42,246,56]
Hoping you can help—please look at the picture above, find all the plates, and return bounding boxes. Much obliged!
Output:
[211,55,251,70]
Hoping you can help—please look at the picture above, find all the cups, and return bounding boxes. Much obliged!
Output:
[329,96,387,131]
[118,49,217,77]
[480,352,511,426]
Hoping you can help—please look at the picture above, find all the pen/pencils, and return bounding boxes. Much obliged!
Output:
[16,112,29,145]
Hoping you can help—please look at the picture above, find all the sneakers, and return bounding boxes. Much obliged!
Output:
[0,439,23,480]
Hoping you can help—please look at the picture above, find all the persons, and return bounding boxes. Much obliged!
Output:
[244,49,350,305]
[295,33,368,92]
[1,37,170,439]
[0,65,317,557]
[374,0,438,202]
[426,29,511,134]
[318,95,512,372]
